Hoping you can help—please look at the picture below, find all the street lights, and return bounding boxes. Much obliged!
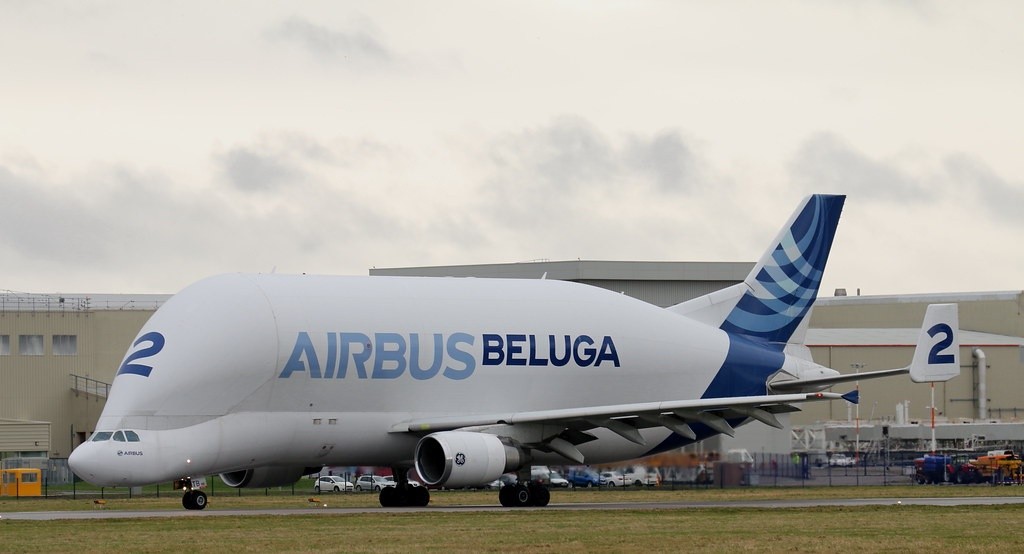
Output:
[850,362,869,465]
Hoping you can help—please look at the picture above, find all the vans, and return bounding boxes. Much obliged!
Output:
[622,465,662,486]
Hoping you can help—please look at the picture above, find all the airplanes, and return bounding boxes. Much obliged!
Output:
[67,194,964,507]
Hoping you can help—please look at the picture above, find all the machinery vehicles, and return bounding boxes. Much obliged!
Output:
[913,450,1024,488]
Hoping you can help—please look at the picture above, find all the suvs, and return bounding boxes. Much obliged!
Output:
[355,471,396,491]
[829,454,849,466]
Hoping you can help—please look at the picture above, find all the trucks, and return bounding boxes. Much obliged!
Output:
[530,465,568,488]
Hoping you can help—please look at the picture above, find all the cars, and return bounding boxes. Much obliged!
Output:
[314,476,354,493]
[384,476,420,487]
[568,466,607,488]
[598,471,632,488]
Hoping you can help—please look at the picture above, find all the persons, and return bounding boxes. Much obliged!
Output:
[772,458,776,468]
[794,454,799,469]
